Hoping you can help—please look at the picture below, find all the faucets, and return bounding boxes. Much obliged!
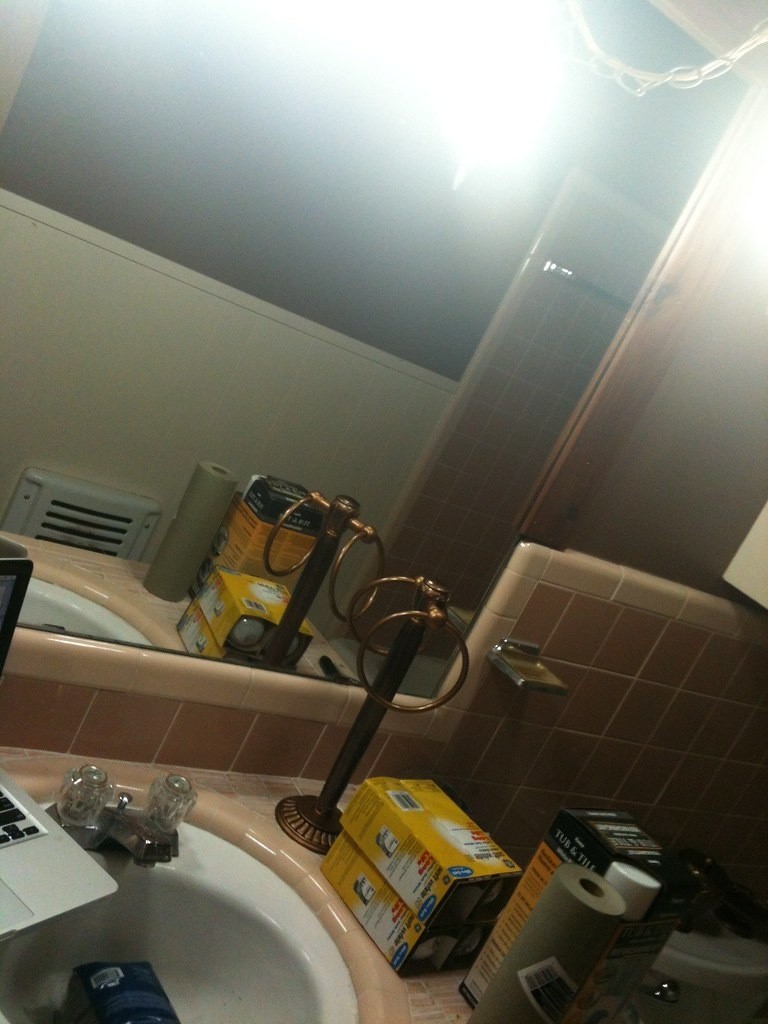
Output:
[96,809,161,872]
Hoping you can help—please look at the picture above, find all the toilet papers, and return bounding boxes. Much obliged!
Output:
[141,458,241,604]
[468,863,618,1021]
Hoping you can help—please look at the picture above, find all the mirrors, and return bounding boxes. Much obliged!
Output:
[0,1,768,700]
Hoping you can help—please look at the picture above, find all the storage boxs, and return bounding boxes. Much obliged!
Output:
[459,808,701,1023]
[176,565,313,669]
[188,477,322,601]
[321,776,524,977]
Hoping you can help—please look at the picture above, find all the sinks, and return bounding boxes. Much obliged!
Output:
[0,569,158,651]
[2,795,359,1024]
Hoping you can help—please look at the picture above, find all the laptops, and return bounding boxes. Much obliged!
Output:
[0,557,119,944]
